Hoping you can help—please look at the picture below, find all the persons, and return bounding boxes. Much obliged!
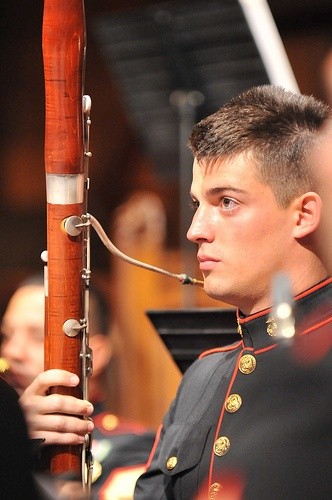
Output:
[1,265,160,499]
[18,81,330,500]
